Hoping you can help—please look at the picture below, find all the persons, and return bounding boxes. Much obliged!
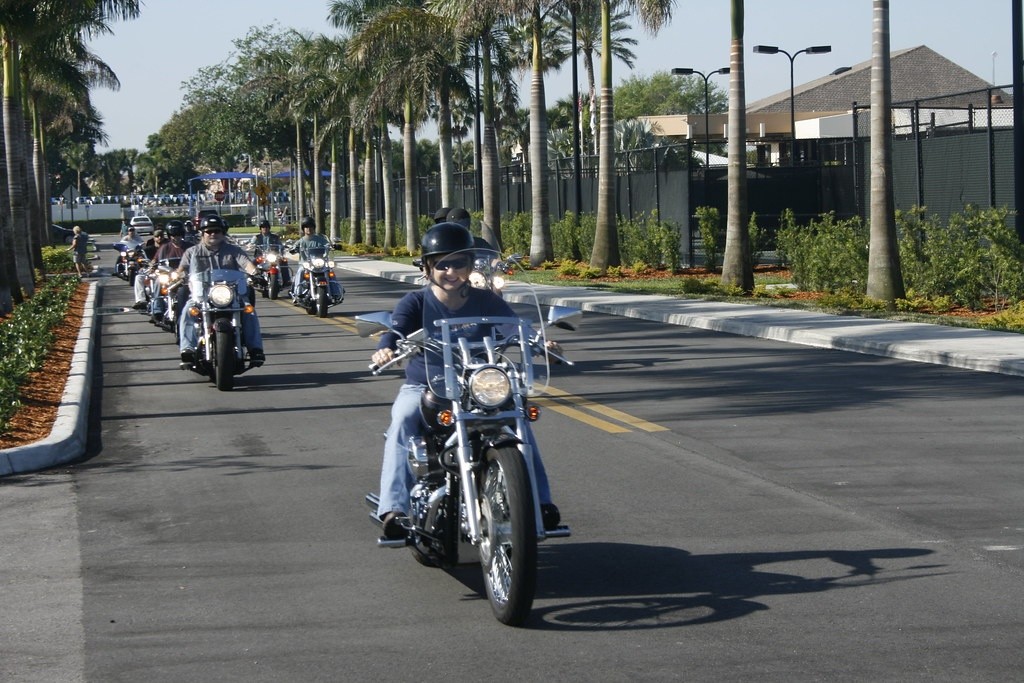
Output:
[249,220,289,287]
[278,206,289,224]
[433,206,502,268]
[115,214,265,364]
[291,216,344,304]
[65,226,91,278]
[371,222,563,540]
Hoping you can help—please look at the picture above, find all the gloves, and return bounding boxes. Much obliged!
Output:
[253,274,268,287]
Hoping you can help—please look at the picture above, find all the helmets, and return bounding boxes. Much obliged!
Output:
[301,217,316,230]
[200,214,224,229]
[446,208,470,226]
[421,223,474,266]
[434,208,449,223]
[259,220,269,227]
[165,220,185,239]
[184,220,194,226]
[128,227,135,232]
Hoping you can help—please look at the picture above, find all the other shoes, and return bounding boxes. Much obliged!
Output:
[181,349,195,362]
[251,348,265,361]
[543,504,560,530]
[383,511,408,539]
[149,313,163,323]
[132,301,146,309]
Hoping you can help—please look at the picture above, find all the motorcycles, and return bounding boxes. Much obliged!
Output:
[137,257,188,345]
[245,239,295,300]
[111,234,241,287]
[354,247,585,625]
[166,265,269,392]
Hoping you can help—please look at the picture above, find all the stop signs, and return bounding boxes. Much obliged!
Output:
[215,191,226,202]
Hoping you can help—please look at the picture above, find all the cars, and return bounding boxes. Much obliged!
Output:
[129,216,155,235]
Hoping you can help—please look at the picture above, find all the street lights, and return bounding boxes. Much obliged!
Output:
[670,67,730,208]
[753,44,832,167]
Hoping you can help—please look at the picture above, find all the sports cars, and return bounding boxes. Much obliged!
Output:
[286,239,346,318]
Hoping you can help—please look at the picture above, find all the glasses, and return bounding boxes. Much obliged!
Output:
[203,229,223,234]
[433,258,468,270]
[155,234,163,237]
